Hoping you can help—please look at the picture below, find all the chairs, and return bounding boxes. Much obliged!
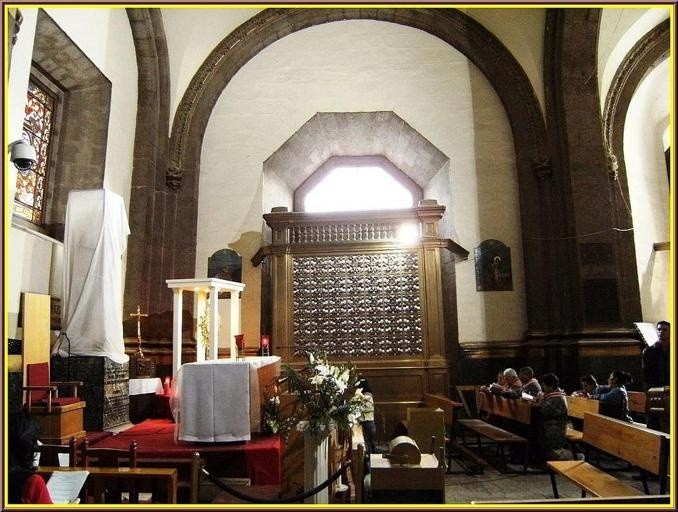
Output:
[34,435,140,503]
[21,289,86,443]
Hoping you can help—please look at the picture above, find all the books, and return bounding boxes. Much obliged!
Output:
[46,469,89,504]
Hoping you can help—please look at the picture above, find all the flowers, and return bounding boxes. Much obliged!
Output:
[283,349,368,446]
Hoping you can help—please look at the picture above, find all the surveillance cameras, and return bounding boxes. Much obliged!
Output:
[11,140,38,170]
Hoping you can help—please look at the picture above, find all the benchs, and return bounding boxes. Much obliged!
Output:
[402,384,672,498]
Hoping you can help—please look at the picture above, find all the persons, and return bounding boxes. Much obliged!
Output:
[353,377,375,454]
[641,321,670,481]
[481,366,633,469]
[8,412,51,503]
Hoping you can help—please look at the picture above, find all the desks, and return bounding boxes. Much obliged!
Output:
[175,356,281,443]
[34,465,178,503]
